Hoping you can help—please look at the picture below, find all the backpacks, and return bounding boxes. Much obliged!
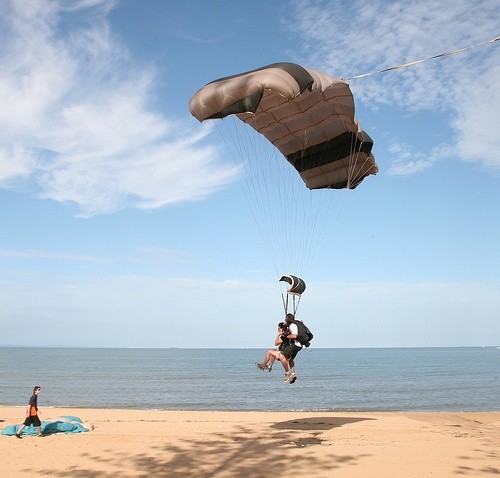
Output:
[290,320,313,347]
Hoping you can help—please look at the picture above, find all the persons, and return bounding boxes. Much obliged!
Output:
[279,314,303,384]
[16,386,45,438]
[256,323,292,372]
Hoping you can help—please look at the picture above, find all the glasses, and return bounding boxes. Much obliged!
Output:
[36,389,40,391]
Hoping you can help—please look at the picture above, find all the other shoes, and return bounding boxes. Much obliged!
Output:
[15,434,22,439]
[38,434,47,437]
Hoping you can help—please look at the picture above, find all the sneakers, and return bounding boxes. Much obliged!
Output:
[264,365,272,372]
[256,362,266,370]
[289,374,297,384]
[283,373,292,383]
